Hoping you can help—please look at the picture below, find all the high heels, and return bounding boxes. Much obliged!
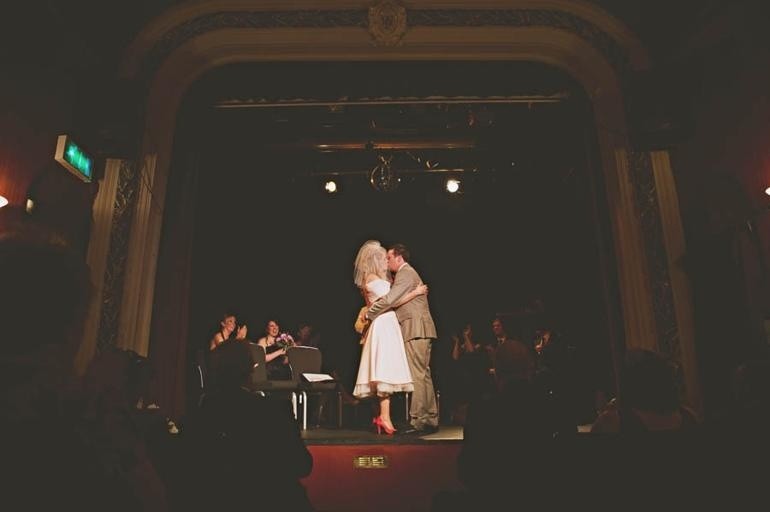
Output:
[372,415,397,435]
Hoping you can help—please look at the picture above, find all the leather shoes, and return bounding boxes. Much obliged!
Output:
[398,426,436,437]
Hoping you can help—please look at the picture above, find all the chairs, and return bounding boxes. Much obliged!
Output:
[243,339,300,423]
[278,340,345,431]
[493,340,531,392]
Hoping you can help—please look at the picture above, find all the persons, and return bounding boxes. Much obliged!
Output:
[360,244,441,437]
[2,343,314,510]
[352,239,430,437]
[212,313,358,430]
[452,315,767,511]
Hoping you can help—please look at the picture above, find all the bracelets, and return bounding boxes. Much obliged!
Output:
[365,312,369,318]
